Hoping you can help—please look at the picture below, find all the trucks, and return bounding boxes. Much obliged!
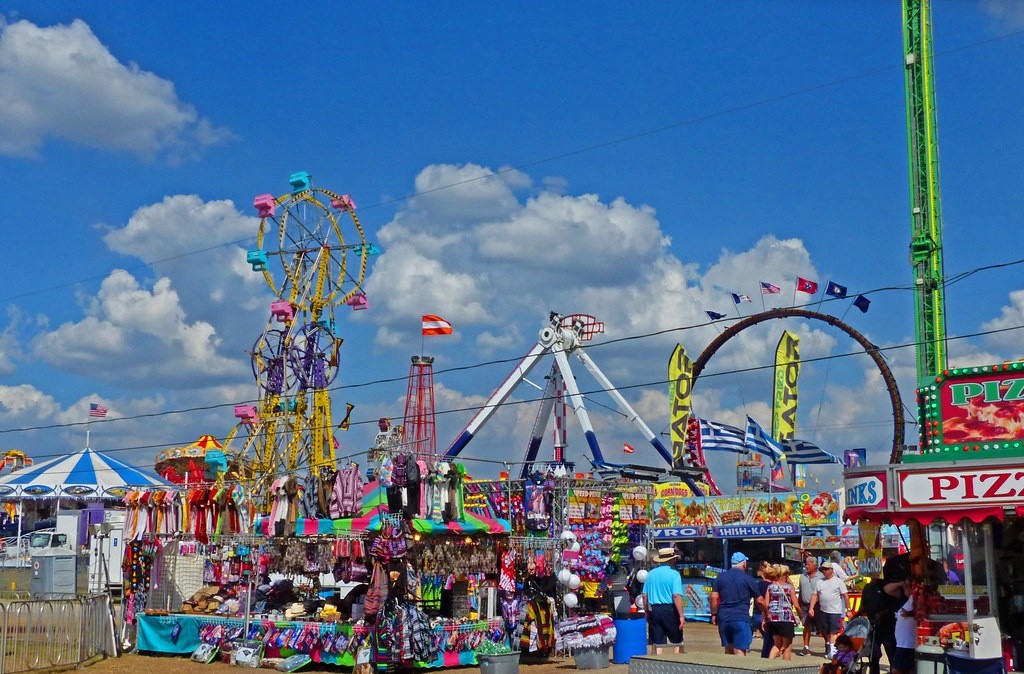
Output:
[88,525,126,594]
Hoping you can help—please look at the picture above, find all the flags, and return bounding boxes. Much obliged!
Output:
[761,282,780,294]
[700,418,748,455]
[797,277,818,295]
[825,280,847,299]
[745,417,784,473]
[422,315,452,335]
[853,294,870,313]
[782,436,848,467]
[732,293,752,304]
[624,442,634,453]
[707,311,727,320]
[89,403,110,417]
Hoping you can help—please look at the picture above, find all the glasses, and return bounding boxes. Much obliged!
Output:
[822,568,832,571]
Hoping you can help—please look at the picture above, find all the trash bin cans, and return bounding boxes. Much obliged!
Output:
[613,611,647,664]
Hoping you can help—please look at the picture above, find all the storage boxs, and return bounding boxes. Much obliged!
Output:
[477,651,521,674]
[572,641,611,669]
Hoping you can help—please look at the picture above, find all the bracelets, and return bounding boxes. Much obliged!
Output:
[848,608,851,611]
[711,614,716,616]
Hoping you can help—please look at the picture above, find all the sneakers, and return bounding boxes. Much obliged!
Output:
[800,647,811,656]
[824,643,831,655]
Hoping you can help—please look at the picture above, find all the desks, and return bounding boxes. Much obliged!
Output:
[135,611,509,669]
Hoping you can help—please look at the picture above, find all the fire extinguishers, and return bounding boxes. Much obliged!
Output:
[1001,632,1015,672]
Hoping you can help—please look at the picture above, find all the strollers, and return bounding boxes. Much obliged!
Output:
[819,616,877,674]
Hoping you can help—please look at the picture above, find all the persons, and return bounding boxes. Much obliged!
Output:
[753,547,1010,674]
[709,551,765,656]
[642,548,686,655]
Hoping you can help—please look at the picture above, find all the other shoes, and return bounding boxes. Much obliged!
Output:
[752,635,761,640]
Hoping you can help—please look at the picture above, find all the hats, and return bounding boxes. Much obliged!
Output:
[821,562,834,569]
[834,635,854,650]
[653,548,679,563]
[731,552,749,564]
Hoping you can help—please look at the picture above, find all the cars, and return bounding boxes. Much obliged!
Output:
[2,531,71,555]
